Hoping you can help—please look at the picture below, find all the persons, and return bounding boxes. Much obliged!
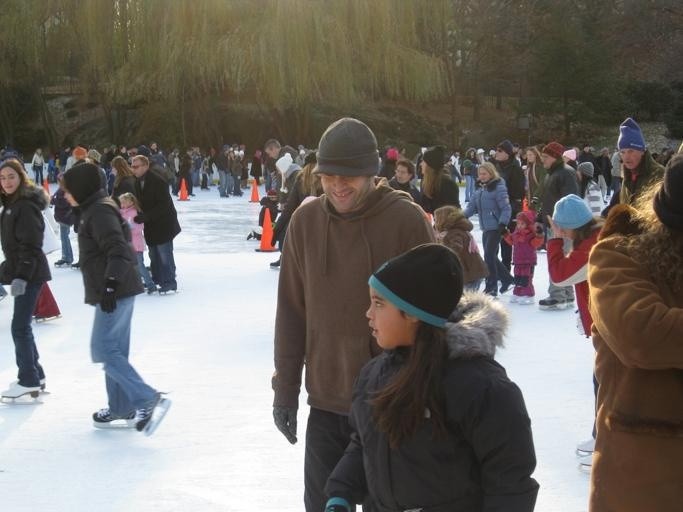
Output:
[0,141,250,437]
[247,117,681,512]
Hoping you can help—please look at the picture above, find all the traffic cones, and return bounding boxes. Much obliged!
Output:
[42,179,51,194]
[522,198,529,213]
[249,180,261,203]
[176,178,190,201]
[255,210,279,255]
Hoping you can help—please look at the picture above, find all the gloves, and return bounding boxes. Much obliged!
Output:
[100,277,120,315]
[9,277,28,298]
[133,210,149,226]
[270,401,300,449]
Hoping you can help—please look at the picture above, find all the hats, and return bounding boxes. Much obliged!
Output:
[61,160,109,206]
[421,145,447,172]
[309,115,383,180]
[475,147,486,155]
[652,153,683,238]
[495,138,514,157]
[386,147,398,162]
[87,148,101,162]
[365,240,466,332]
[541,141,564,161]
[616,115,647,154]
[561,148,577,161]
[72,146,87,161]
[576,161,595,179]
[510,209,535,231]
[550,191,594,232]
[274,151,295,175]
[265,188,280,199]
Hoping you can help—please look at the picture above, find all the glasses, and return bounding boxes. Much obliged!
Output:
[129,164,147,170]
[393,168,410,176]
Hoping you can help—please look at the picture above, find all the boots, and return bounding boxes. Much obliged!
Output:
[1,374,48,408]
[147,287,158,296]
[512,284,536,305]
[481,287,499,299]
[157,285,179,297]
[133,392,174,438]
[245,230,256,241]
[90,406,139,431]
[267,257,282,270]
[499,275,515,295]
[33,305,64,325]
[537,294,578,312]
[603,200,609,205]
[142,276,159,288]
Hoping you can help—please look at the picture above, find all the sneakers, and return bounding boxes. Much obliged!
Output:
[578,439,601,453]
[579,455,592,467]
